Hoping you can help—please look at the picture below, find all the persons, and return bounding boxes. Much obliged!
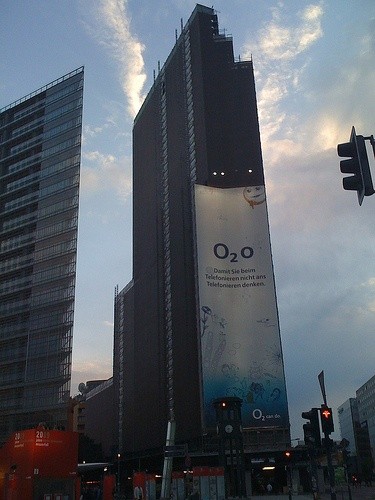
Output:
[260,481,273,495]
[134,483,144,500]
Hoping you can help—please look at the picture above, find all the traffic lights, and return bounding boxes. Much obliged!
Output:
[320,404,335,434]
[334,126,374,206]
[9,463,18,474]
[283,446,293,500]
[301,409,321,437]
